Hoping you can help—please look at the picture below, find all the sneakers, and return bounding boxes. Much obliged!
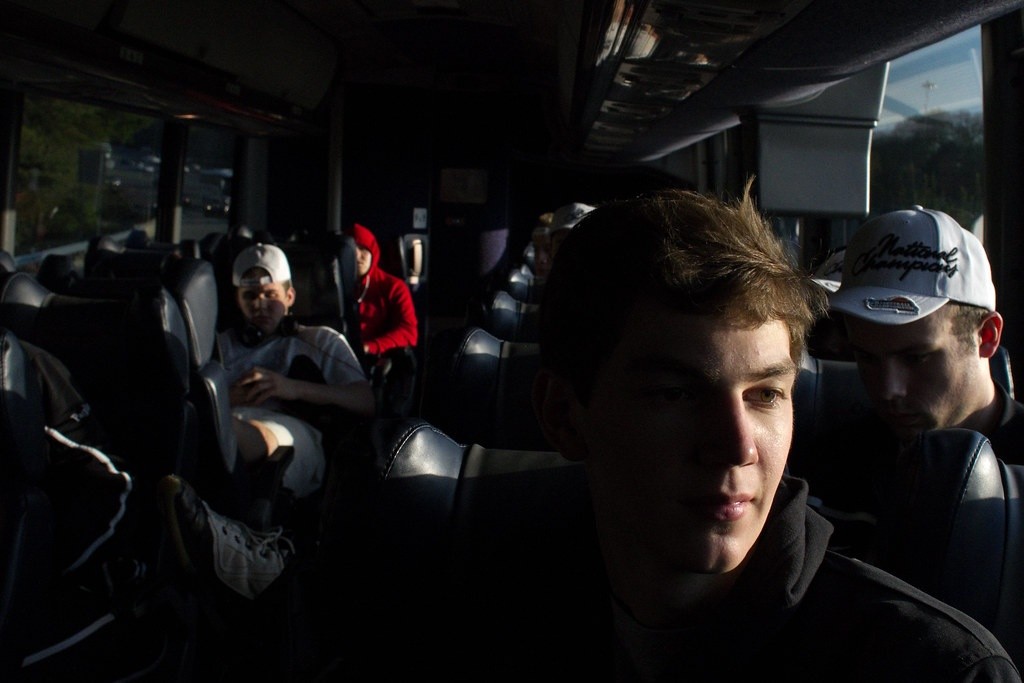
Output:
[168,474,297,603]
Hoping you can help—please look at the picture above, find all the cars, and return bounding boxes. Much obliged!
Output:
[138,154,161,173]
[202,192,231,217]
[151,150,200,175]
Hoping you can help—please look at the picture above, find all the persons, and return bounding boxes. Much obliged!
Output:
[314,172,1024,683]
[0,222,375,683]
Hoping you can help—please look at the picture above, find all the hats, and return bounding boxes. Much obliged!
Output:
[826,204,996,326]
[232,241,292,289]
[809,250,846,294]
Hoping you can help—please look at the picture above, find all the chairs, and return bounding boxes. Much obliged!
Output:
[0,162,1024,681]
